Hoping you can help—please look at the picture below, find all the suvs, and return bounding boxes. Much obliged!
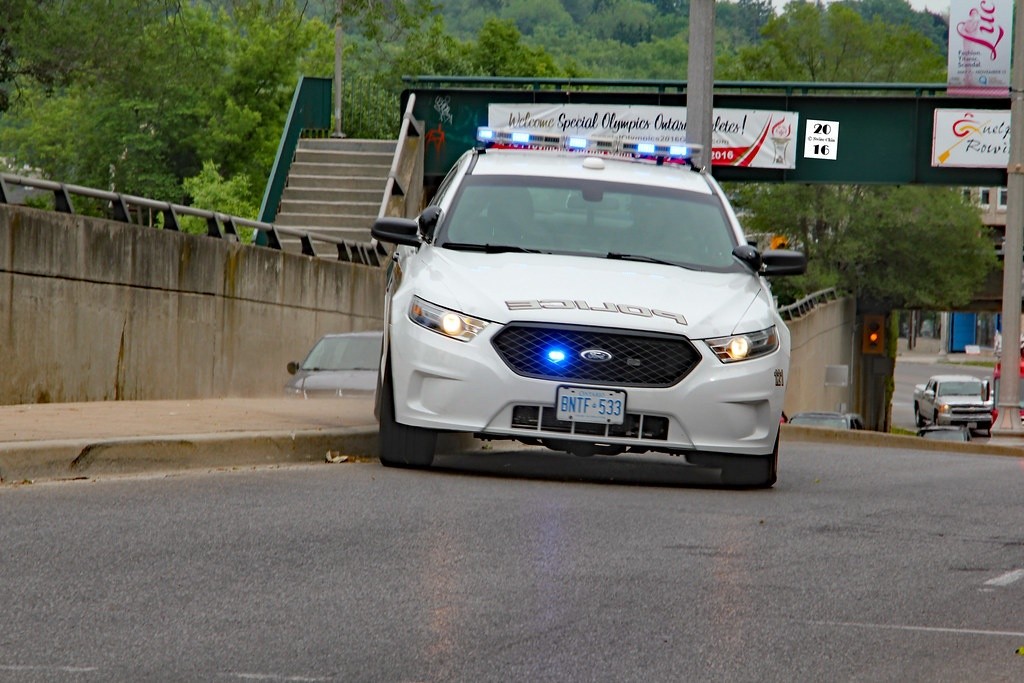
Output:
[915,424,971,443]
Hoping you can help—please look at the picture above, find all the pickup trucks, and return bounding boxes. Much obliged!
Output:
[913,374,993,431]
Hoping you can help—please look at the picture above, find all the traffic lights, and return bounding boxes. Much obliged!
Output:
[862,311,885,354]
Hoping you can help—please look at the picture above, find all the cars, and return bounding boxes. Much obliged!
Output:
[283,330,384,398]
[789,410,866,430]
[370,127,806,489]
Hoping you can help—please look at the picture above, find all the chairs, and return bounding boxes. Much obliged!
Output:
[472,185,552,249]
